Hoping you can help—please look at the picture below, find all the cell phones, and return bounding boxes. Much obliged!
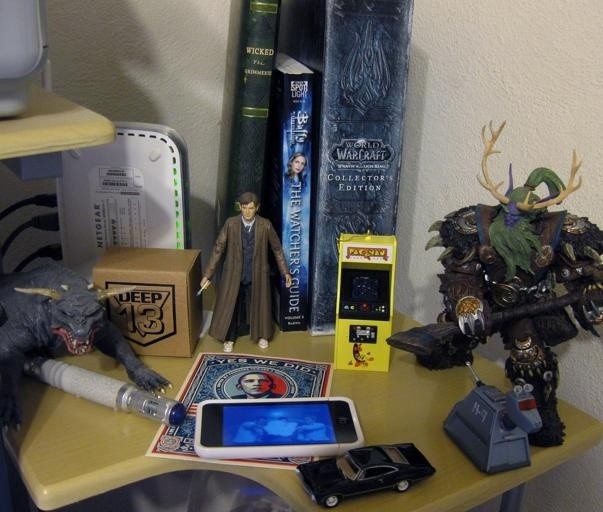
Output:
[194,397,366,462]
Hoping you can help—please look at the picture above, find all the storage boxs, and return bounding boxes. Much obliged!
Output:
[92,247,204,359]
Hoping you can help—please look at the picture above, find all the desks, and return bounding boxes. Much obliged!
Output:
[0,304,602,512]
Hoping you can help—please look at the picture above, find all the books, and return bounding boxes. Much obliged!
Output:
[292,0,416,335]
[214,0,282,339]
[262,53,315,333]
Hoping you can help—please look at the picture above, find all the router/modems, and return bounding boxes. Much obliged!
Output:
[52,121,191,285]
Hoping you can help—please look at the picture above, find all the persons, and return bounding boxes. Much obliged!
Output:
[284,152,310,188]
[230,372,283,400]
[195,190,292,353]
[385,118,602,448]
[232,414,332,441]
